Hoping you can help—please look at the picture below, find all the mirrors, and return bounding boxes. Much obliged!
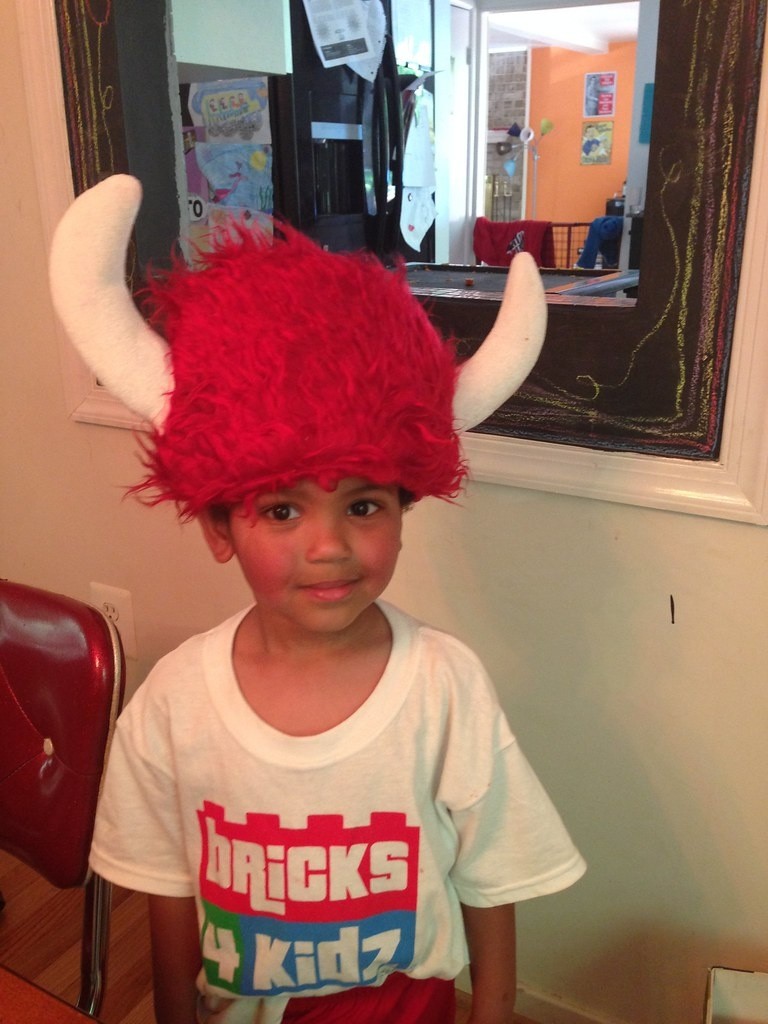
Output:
[52,0,767,465]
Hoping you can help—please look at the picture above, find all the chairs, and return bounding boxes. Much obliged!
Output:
[0,571,128,1024]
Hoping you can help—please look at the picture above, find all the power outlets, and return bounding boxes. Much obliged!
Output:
[87,577,140,664]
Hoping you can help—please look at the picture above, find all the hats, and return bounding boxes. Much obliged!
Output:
[119,215,466,520]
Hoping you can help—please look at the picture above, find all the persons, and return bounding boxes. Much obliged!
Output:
[49,172,589,1023]
